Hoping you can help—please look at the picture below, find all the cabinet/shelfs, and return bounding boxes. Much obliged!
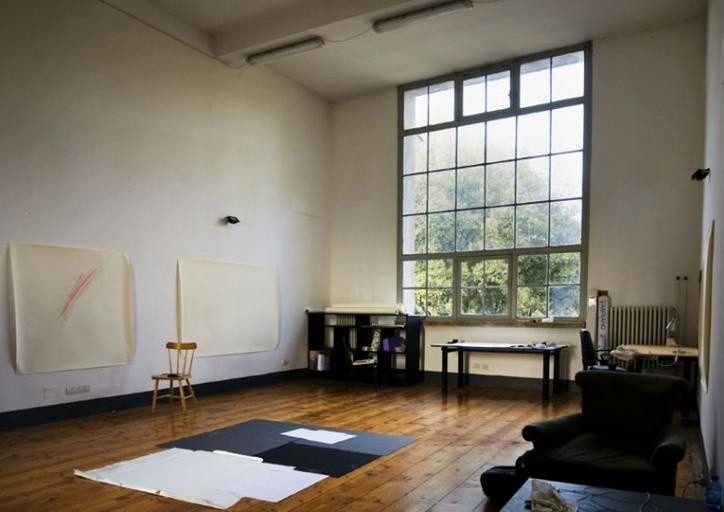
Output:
[306,308,422,387]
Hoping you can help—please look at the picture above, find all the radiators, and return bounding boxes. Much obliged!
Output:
[606,307,672,371]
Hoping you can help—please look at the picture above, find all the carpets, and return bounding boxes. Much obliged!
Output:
[66,418,421,510]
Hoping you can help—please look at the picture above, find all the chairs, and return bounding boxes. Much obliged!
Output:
[577,326,619,371]
[515,371,690,495]
[151,343,197,411]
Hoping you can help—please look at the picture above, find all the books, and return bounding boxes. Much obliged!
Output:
[308,314,357,371]
[369,315,407,326]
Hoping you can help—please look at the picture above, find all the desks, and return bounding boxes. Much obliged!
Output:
[619,342,698,377]
[432,341,568,406]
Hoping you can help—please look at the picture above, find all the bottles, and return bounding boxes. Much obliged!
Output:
[666,316,677,345]
